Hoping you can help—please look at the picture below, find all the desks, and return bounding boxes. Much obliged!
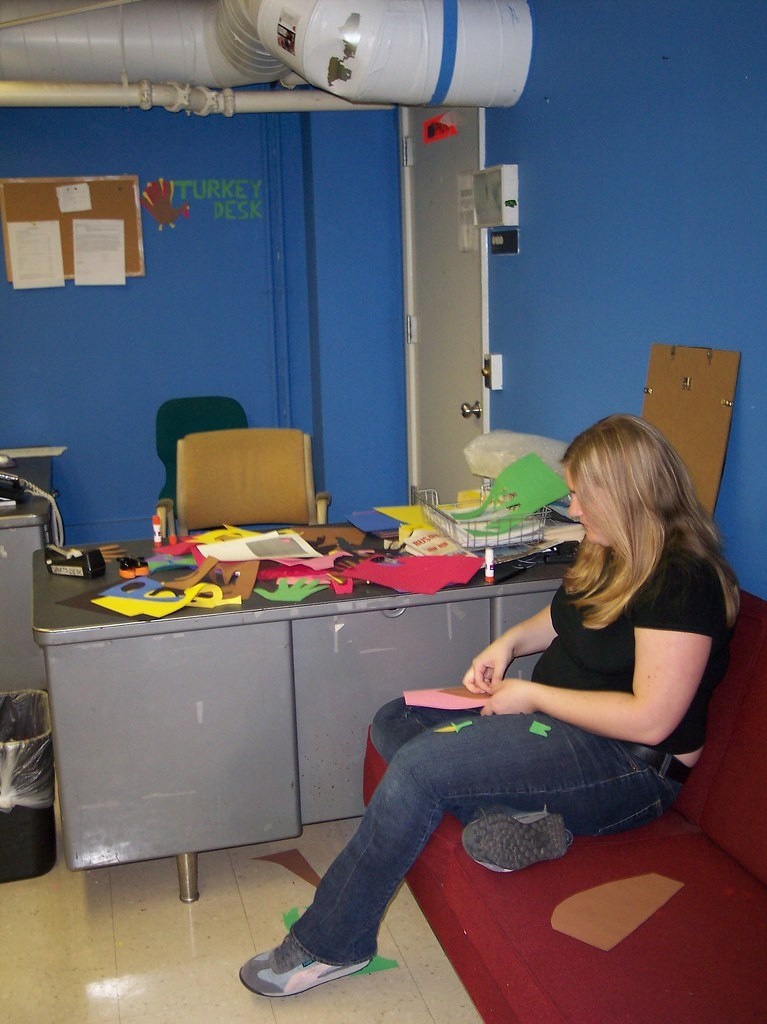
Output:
[0,456,52,692]
[33,521,576,903]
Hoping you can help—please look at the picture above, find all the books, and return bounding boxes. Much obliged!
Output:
[537,523,586,541]
[434,501,545,549]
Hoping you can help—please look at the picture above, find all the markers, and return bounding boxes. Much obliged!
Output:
[97,544,127,563]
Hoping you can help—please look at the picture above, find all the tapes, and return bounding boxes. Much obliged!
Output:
[48,545,83,559]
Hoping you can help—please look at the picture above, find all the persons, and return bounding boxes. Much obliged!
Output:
[240,413,740,998]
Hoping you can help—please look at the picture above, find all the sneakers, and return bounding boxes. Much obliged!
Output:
[239,932,372,997]
[461,805,568,872]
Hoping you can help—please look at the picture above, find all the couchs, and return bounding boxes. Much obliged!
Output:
[362,588,767,1024]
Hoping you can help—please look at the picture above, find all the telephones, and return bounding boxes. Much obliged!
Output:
[0,472,24,499]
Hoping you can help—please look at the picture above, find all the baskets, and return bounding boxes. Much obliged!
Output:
[416,482,552,552]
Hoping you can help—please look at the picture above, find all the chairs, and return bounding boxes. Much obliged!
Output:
[176,428,331,536]
[155,396,248,539]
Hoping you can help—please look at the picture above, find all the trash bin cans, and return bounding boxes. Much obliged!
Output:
[0,685,61,889]
